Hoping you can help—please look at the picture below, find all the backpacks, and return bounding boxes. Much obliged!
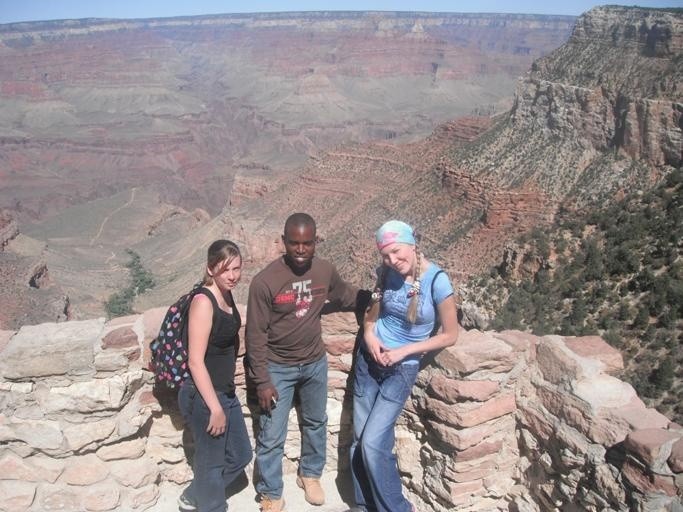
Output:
[149,287,192,391]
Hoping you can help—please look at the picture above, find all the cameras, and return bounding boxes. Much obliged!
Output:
[270,394,277,411]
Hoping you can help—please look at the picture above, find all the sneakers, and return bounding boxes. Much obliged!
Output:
[295,476,327,506]
[260,494,286,512]
[179,494,229,512]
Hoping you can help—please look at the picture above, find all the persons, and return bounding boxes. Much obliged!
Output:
[174,240,254,512]
[343,216,459,510]
[240,212,380,511]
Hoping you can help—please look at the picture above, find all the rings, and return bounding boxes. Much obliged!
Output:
[384,360,390,364]
